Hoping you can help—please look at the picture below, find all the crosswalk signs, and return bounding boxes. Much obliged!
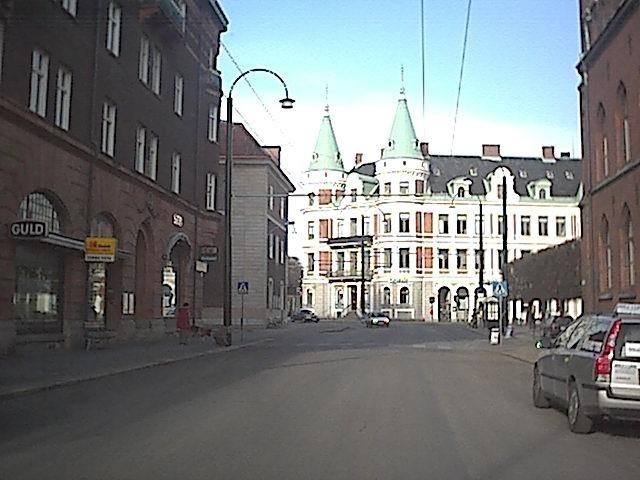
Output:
[237,281,249,294]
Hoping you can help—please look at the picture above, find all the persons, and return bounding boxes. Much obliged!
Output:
[176,302,192,346]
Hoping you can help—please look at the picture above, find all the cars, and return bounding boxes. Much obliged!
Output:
[292,309,320,323]
[532,302,640,435]
[368,311,389,329]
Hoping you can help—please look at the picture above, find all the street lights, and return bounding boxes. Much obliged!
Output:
[449,157,509,335]
[361,205,388,314]
[222,67,295,347]
